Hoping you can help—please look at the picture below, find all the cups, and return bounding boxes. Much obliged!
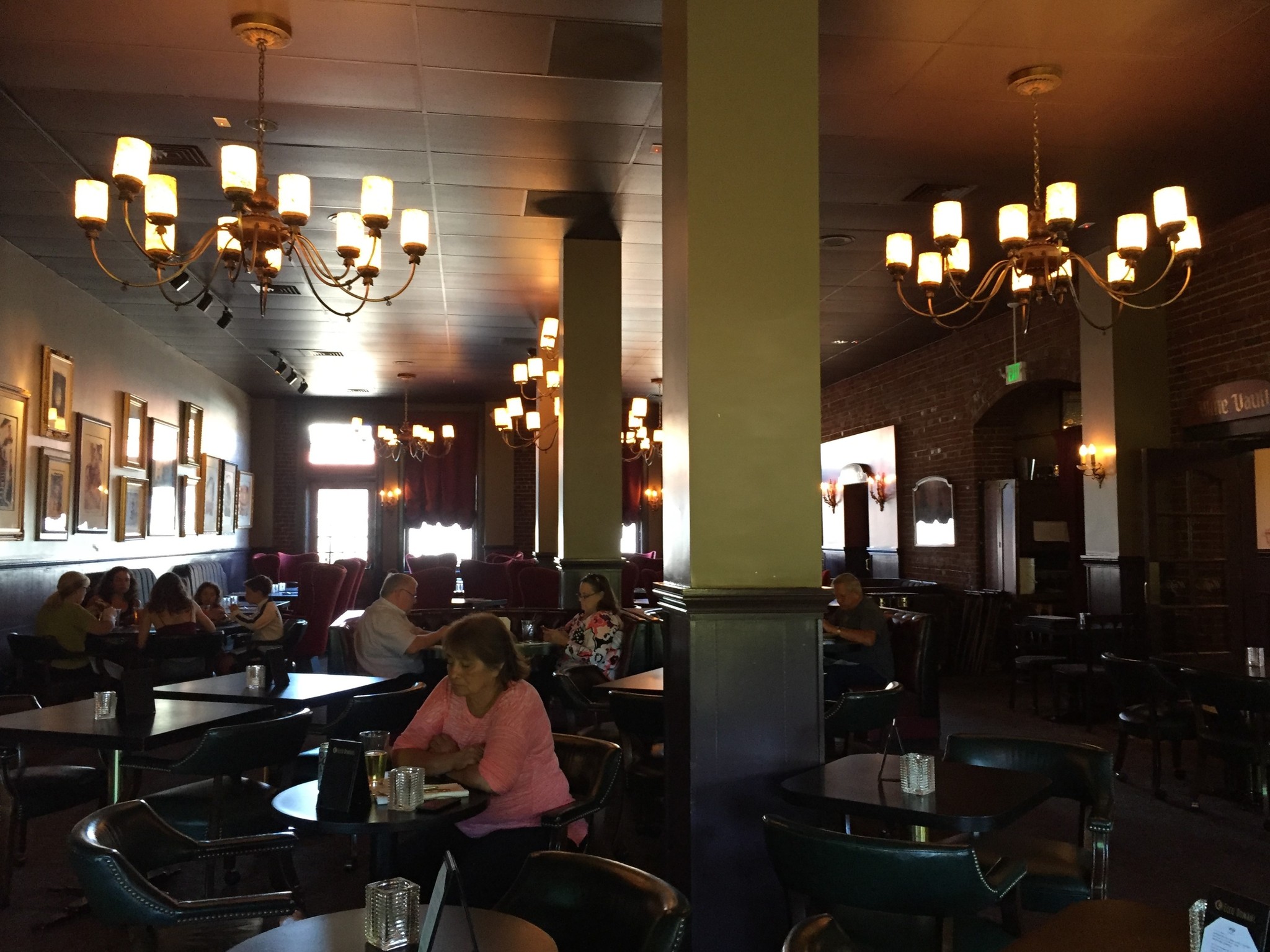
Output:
[365,875,421,952]
[899,753,937,795]
[1246,647,1264,666]
[245,665,266,688]
[219,596,238,613]
[388,766,425,813]
[94,691,117,720]
[522,620,534,644]
[271,583,287,593]
[360,731,390,788]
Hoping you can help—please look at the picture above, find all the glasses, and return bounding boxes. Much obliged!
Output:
[82,586,91,593]
[401,587,417,599]
[575,591,597,599]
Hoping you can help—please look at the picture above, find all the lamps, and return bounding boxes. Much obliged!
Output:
[378,488,401,517]
[1076,443,1106,489]
[885,62,1201,336]
[867,473,889,512]
[349,373,454,462]
[75,9,433,328]
[495,316,560,452]
[620,377,662,465]
[820,478,837,514]
[644,488,663,512]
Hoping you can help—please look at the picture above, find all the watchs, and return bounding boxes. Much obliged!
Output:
[836,626,841,636]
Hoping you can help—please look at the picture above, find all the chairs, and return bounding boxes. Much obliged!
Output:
[0,554,1270,952]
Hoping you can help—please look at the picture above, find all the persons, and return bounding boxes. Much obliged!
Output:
[822,573,894,738]
[138,573,216,686]
[85,567,141,661]
[392,612,586,909]
[33,571,117,696]
[353,573,449,724]
[531,574,625,729]
[193,581,225,678]
[217,574,284,675]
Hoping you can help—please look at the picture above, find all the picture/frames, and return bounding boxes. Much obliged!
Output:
[0,341,257,543]
